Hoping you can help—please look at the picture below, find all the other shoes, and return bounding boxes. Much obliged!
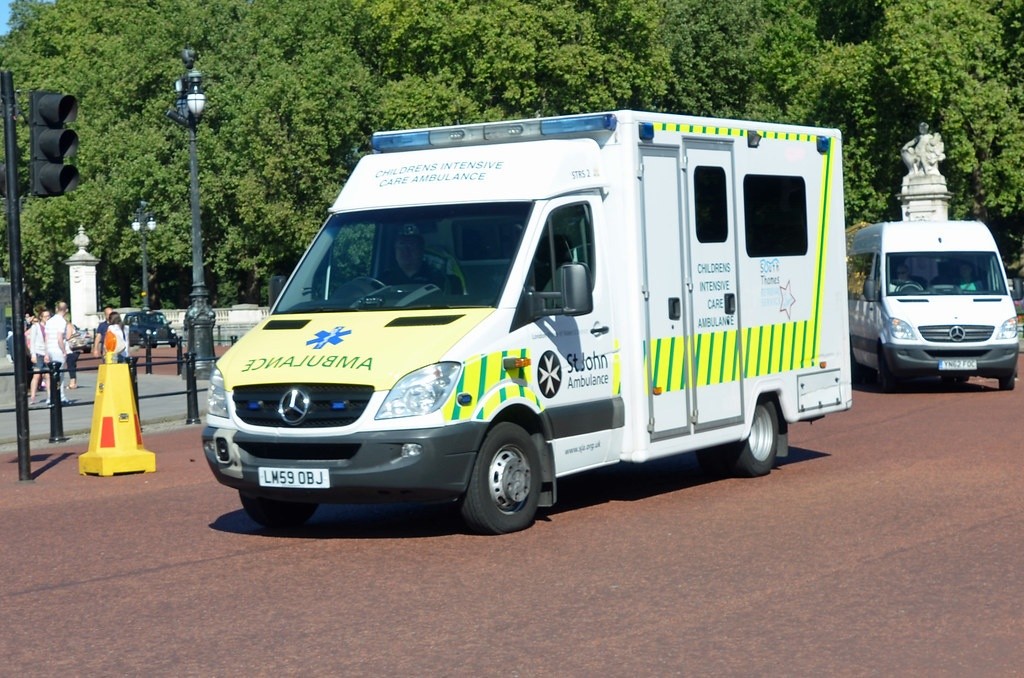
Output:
[47,398,75,407]
[30,397,39,405]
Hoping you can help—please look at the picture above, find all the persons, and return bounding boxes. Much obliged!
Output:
[376,234,454,294]
[902,122,933,175]
[94,308,114,362]
[953,262,986,292]
[28,306,53,406]
[890,264,917,292]
[44,301,72,406]
[103,311,130,363]
[5,310,83,391]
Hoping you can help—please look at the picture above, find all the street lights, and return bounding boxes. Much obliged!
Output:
[129,199,157,309]
[167,48,215,424]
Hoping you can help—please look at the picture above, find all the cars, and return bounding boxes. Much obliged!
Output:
[123,310,179,349]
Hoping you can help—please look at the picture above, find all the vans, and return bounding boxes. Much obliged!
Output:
[847,220,1020,394]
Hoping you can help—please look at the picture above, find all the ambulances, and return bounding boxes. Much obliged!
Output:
[199,109,852,533]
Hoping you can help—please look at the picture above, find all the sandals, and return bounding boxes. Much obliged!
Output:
[66,383,79,390]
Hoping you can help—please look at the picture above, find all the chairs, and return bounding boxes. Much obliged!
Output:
[533,233,574,292]
[424,245,466,296]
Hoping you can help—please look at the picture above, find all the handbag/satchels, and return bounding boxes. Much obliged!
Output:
[65,324,87,351]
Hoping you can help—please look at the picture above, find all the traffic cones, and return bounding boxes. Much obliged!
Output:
[76,323,157,480]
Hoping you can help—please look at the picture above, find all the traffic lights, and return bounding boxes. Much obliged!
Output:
[27,91,79,198]
